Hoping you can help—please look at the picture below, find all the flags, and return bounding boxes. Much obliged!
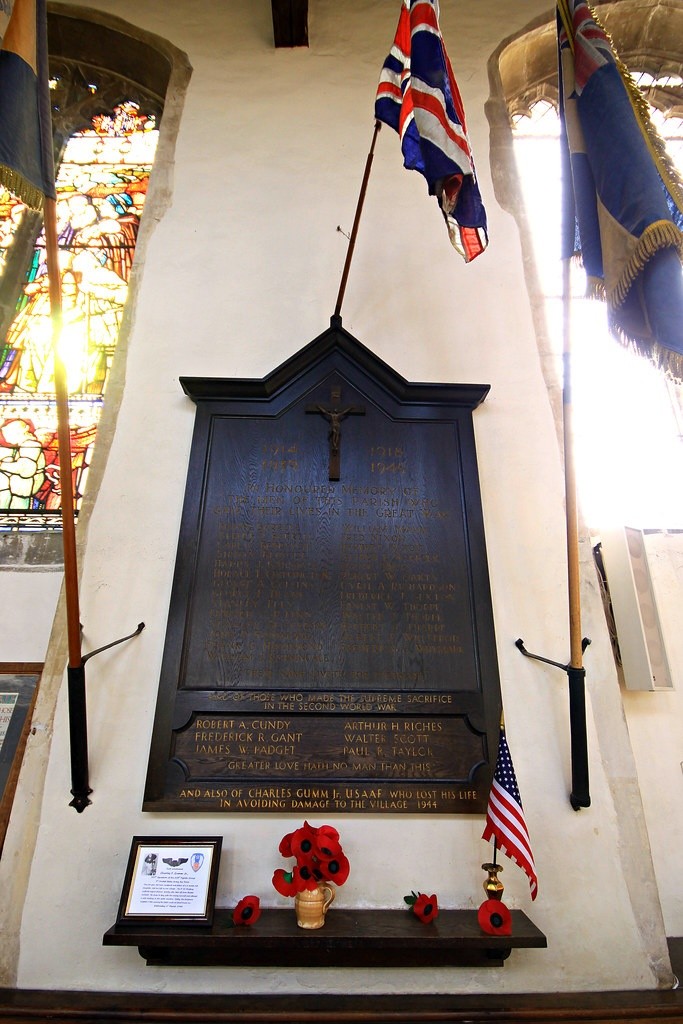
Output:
[482,725,539,901]
[376,0,492,263]
[558,1,682,388]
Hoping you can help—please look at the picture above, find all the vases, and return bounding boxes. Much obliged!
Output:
[295,882,336,929]
[481,863,504,901]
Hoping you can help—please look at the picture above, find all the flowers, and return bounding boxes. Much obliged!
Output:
[272,820,350,897]
[478,900,512,936]
[229,895,260,923]
[404,891,439,922]
